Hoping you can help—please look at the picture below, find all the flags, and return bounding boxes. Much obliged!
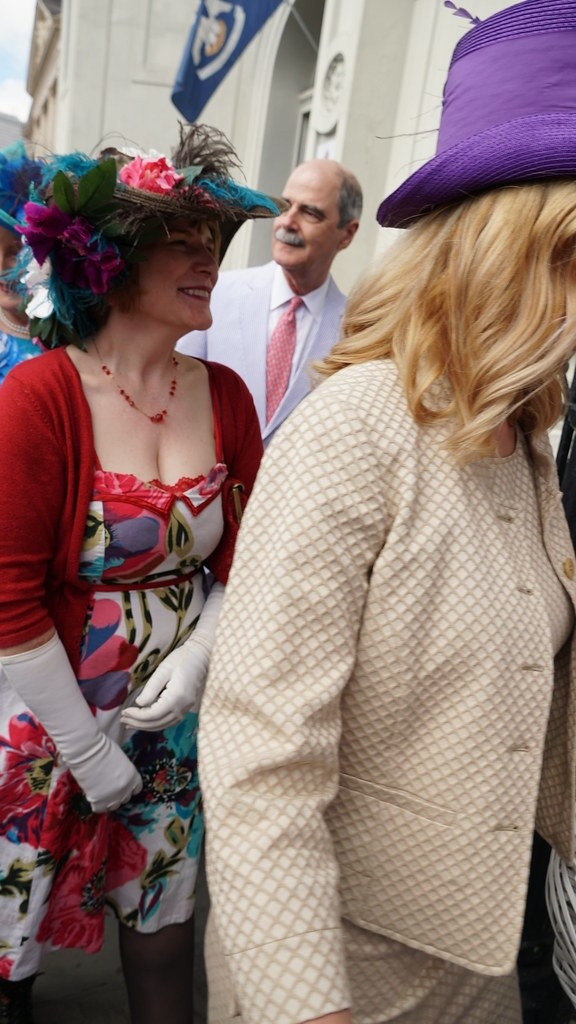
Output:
[171,0,283,123]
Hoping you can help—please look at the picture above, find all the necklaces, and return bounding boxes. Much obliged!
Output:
[1,311,31,335]
[93,338,179,423]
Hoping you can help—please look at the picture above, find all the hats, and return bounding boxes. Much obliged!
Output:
[0,116,294,348]
[376,1,576,230]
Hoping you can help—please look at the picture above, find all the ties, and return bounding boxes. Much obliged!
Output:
[264,296,305,423]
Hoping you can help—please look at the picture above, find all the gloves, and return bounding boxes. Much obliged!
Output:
[121,582,227,732]
[1,630,143,813]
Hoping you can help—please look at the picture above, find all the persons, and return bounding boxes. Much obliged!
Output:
[172,157,364,454]
[197,0,576,1024]
[1,120,288,1024]
[0,142,98,381]
